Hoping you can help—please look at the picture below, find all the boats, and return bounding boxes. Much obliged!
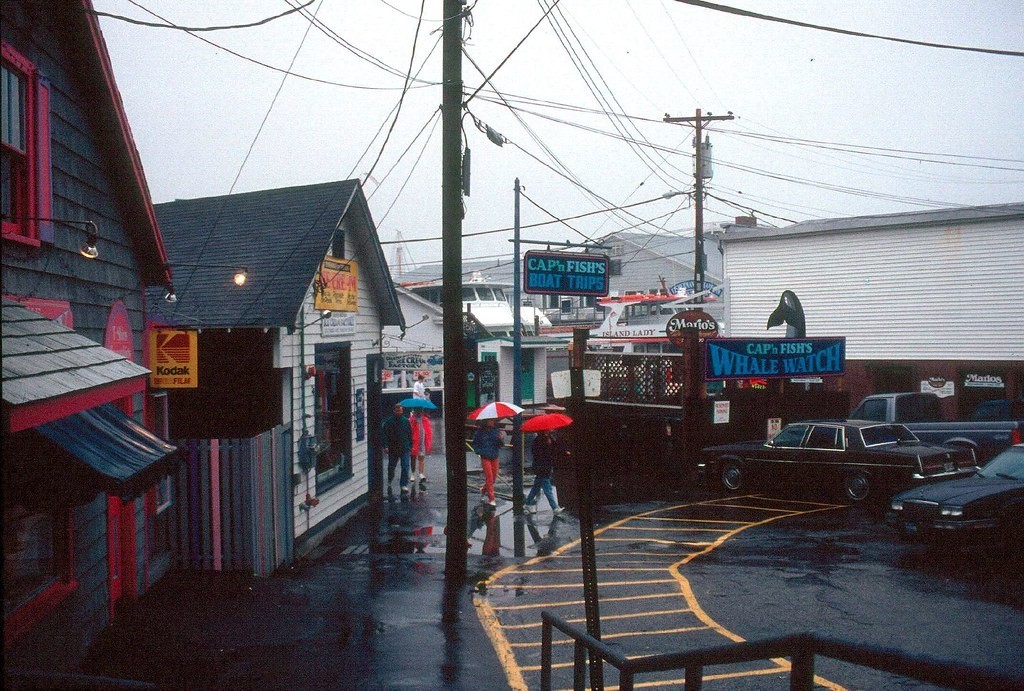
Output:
[400,269,554,328]
[565,276,725,351]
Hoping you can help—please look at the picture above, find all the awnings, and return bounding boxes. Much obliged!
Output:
[0,295,186,504]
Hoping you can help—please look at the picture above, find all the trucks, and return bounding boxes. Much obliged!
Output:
[844,390,1024,465]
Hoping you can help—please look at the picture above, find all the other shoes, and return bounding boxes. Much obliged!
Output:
[388,483,393,490]
[401,486,408,491]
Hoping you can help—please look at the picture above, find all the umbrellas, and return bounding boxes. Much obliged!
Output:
[399,399,437,410]
[466,401,525,434]
[520,413,573,433]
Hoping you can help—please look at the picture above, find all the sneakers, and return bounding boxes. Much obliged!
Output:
[418,474,426,483]
[553,507,565,515]
[487,498,497,507]
[410,473,415,482]
[523,503,537,514]
[479,487,485,501]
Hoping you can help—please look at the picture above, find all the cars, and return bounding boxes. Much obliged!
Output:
[882,442,1024,535]
[695,418,982,506]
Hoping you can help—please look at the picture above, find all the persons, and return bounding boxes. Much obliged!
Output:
[471,419,505,506]
[522,429,571,515]
[413,375,432,418]
[382,406,432,492]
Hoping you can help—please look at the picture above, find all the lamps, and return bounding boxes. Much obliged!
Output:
[0,213,100,259]
[287,310,332,336]
[165,287,179,302]
[401,313,429,329]
[163,261,249,287]
